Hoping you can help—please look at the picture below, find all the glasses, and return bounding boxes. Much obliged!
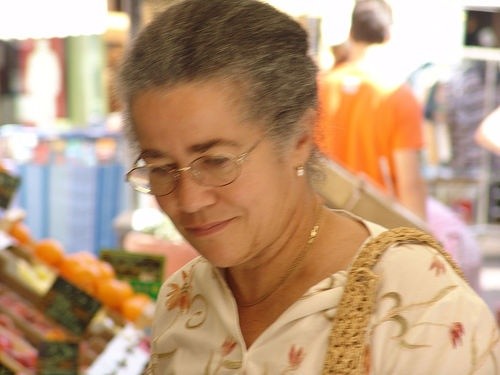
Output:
[124,123,271,197]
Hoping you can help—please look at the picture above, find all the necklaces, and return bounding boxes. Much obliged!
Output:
[230,207,323,307]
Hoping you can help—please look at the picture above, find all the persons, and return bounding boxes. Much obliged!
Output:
[313,0,427,221]
[118,0,500,375]
